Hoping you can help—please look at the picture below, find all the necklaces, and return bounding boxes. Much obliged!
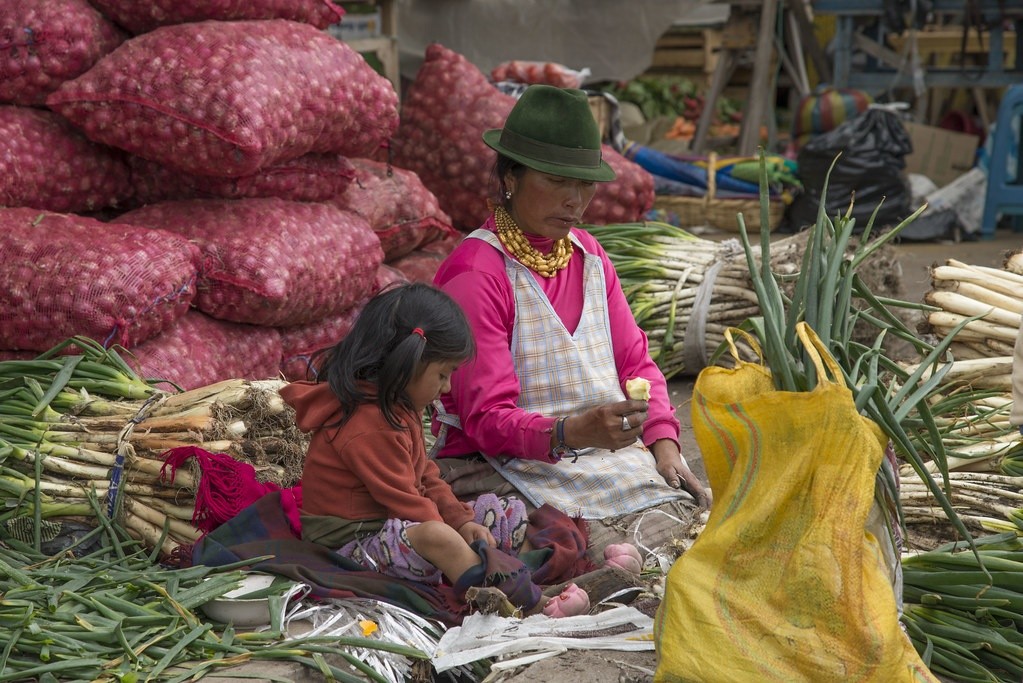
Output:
[494,205,574,278]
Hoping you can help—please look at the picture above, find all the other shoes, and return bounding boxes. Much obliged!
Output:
[536,582,591,619]
[602,543,643,580]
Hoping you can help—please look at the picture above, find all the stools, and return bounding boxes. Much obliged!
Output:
[980,85,1023,240]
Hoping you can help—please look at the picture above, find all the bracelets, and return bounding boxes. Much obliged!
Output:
[552,416,578,463]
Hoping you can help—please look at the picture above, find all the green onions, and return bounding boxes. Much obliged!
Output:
[0,332,435,683]
[571,144,1023,683]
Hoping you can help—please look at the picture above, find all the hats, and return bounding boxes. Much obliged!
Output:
[482,84,617,182]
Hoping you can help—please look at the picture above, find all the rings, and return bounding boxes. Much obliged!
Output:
[621,417,630,431]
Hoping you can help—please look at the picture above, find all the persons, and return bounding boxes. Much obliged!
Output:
[426,83,709,520]
[277,282,553,620]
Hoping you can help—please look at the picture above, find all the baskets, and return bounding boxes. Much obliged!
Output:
[654,151,784,233]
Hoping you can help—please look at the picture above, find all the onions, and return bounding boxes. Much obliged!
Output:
[0,0,659,394]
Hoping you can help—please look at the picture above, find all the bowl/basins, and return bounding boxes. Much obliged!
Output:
[197,573,303,629]
